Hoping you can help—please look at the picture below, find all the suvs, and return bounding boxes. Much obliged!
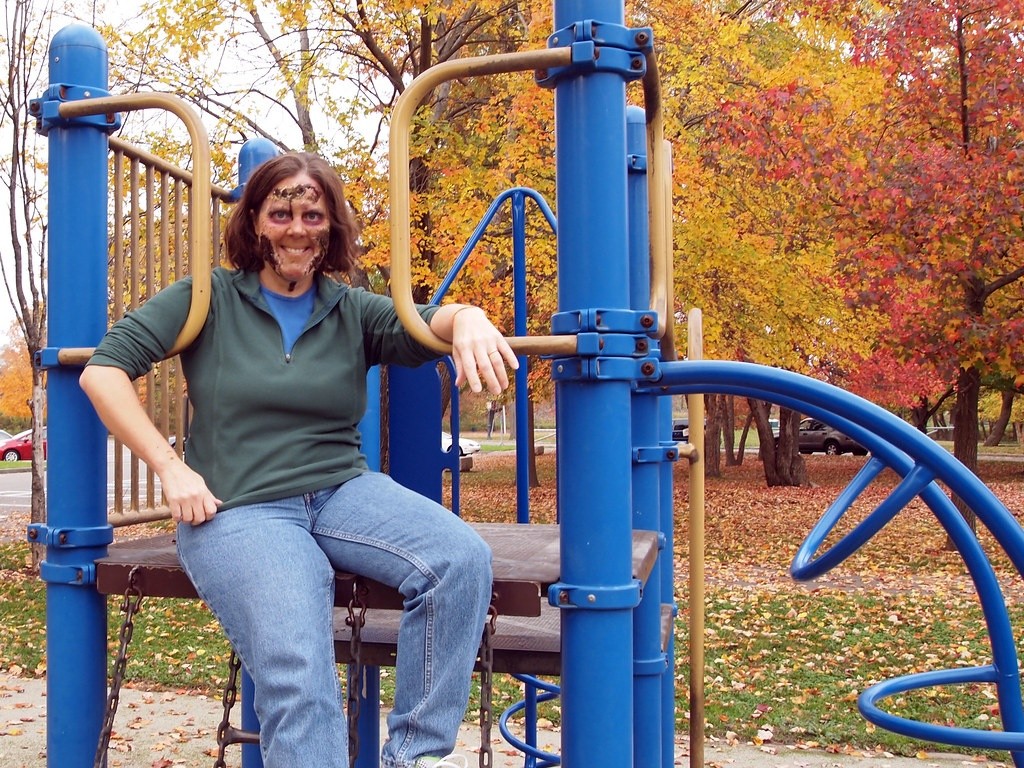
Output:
[773,417,868,456]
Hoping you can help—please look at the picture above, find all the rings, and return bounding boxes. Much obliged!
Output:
[488,349,498,356]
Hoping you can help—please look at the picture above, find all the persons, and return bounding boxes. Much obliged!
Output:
[78,150,525,768]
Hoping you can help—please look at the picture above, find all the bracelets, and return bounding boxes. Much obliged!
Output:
[450,305,484,326]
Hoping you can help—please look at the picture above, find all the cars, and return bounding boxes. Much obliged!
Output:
[672,419,707,441]
[768,418,780,438]
[0,426,47,462]
[0,430,12,443]
[441,431,481,456]
[169,436,186,451]
